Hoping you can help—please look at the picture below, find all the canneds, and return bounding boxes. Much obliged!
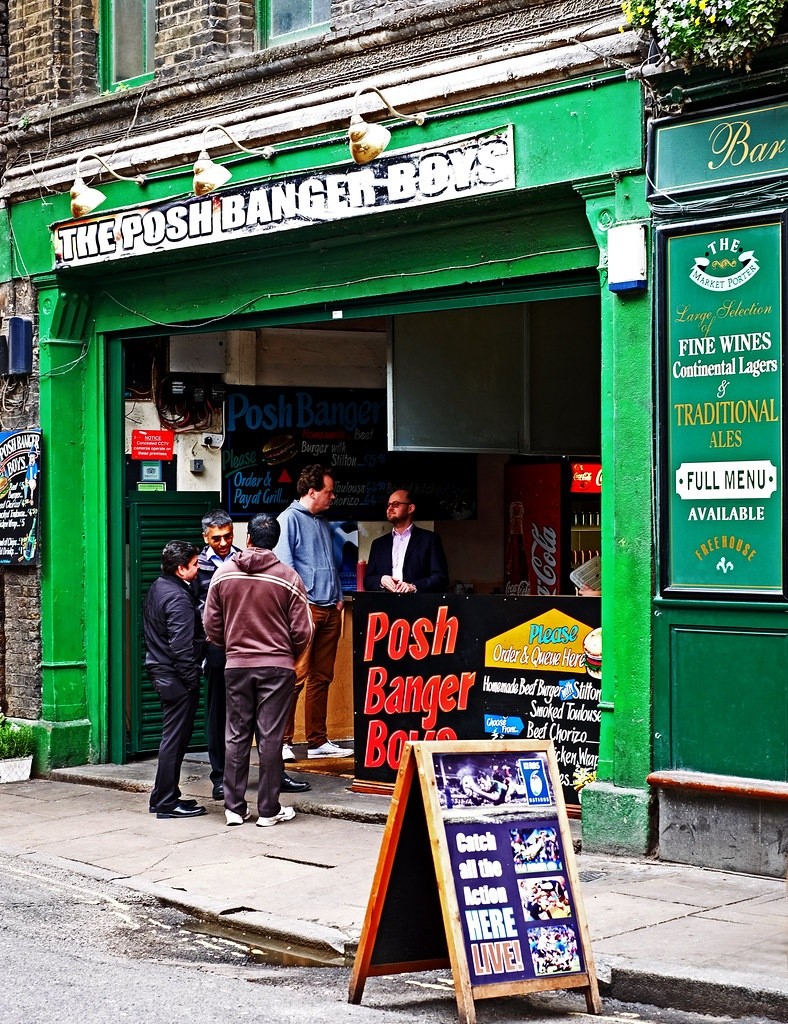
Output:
[572,510,601,565]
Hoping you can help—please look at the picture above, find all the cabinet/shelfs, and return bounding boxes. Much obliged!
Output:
[291,595,354,744]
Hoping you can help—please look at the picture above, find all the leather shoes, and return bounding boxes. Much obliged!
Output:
[279,777,311,792]
[213,783,225,800]
[149,799,207,819]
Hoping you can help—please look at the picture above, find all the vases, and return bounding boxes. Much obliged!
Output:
[624,33,788,95]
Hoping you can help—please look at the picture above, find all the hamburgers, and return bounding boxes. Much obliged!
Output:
[263,434,298,466]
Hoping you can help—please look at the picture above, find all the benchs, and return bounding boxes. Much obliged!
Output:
[645,770,787,880]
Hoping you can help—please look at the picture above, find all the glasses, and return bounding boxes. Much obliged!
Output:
[384,501,409,508]
[212,533,233,541]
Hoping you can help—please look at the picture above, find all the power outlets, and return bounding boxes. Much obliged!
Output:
[201,433,222,447]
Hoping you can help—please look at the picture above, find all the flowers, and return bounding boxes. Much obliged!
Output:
[617,0,788,76]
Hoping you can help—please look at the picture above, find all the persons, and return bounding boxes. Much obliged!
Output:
[579,584,601,596]
[271,465,354,762]
[143,539,205,818]
[194,509,312,799]
[363,490,450,594]
[202,514,316,827]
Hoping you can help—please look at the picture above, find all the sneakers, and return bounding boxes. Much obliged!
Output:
[281,743,296,761]
[256,805,296,827]
[307,739,354,759]
[224,807,251,826]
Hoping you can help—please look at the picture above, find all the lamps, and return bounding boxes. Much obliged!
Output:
[191,125,276,199]
[7,316,27,375]
[70,151,149,219]
[348,85,427,165]
